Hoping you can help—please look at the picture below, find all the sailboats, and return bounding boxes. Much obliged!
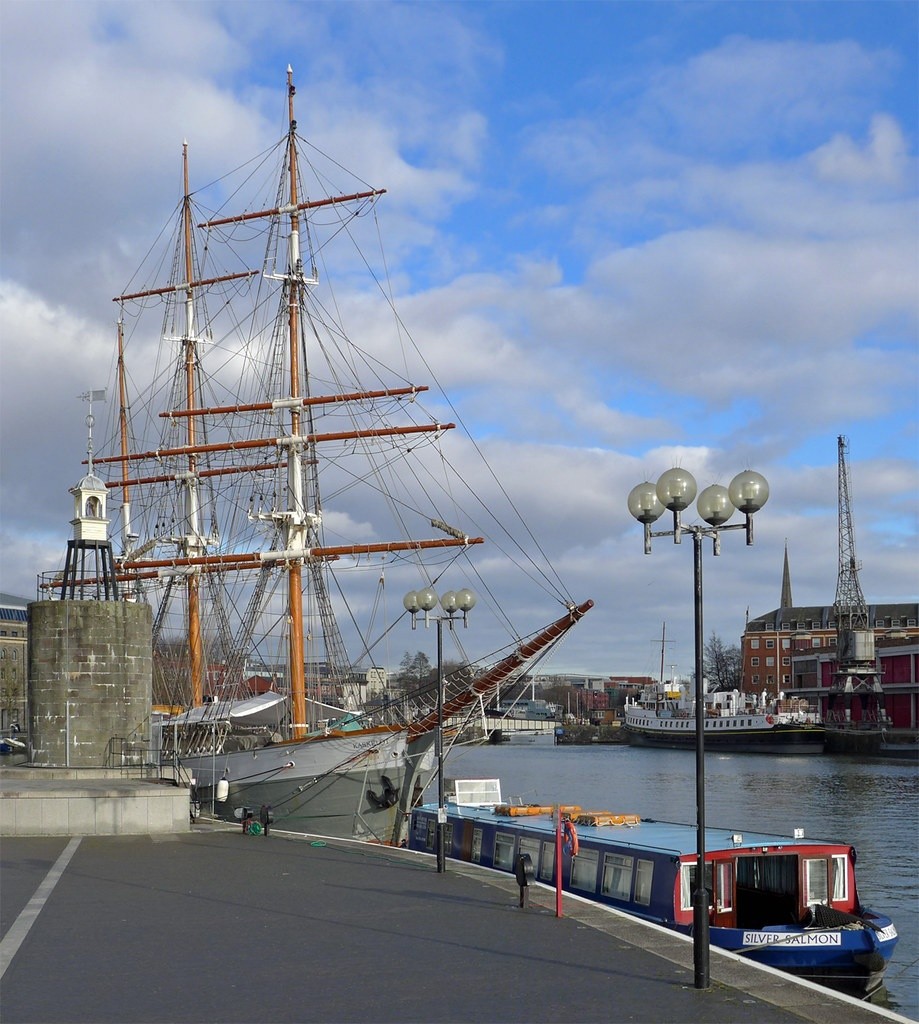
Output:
[38,64,597,850]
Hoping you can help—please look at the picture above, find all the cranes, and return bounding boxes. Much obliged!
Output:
[830,433,888,731]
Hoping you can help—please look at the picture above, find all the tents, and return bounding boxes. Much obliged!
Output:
[302,713,363,737]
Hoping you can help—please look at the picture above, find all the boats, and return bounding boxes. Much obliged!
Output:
[621,664,829,755]
[403,794,898,1000]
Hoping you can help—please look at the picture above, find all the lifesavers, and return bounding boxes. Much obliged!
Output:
[564,822,578,857]
[766,715,774,724]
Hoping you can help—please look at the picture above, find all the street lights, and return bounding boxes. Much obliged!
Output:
[625,466,768,994]
[402,588,474,880]
[65,688,80,767]
